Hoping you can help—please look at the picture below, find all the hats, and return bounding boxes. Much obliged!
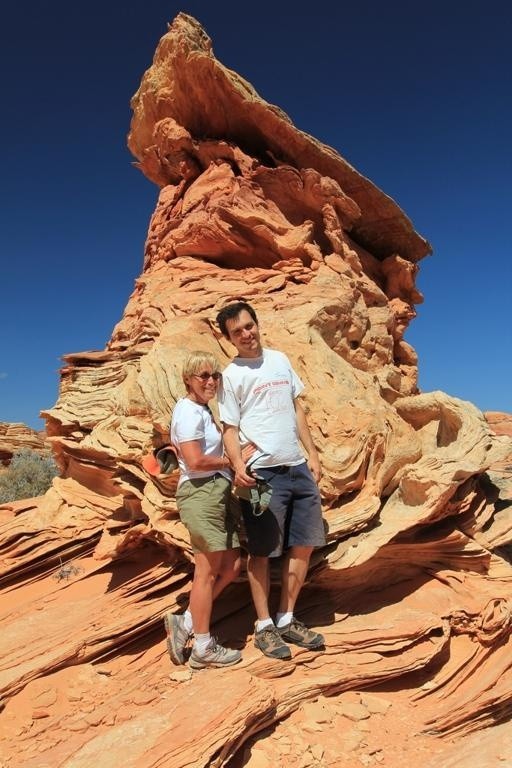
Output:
[152,442,181,474]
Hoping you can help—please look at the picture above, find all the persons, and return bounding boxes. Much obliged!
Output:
[214,301,326,659]
[165,353,242,669]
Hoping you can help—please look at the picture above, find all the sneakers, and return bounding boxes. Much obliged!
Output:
[163,613,195,666]
[252,622,292,659]
[187,636,242,668]
[275,617,326,649]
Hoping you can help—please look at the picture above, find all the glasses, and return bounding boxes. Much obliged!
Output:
[245,453,288,487]
[191,372,223,380]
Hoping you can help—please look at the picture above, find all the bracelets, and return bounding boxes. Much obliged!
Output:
[218,456,225,470]
